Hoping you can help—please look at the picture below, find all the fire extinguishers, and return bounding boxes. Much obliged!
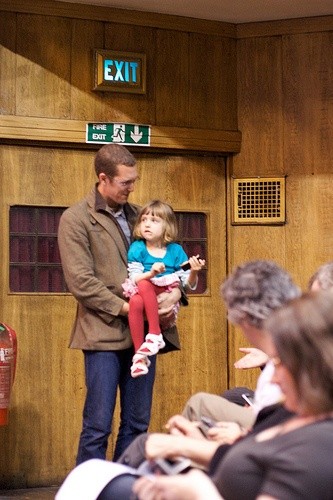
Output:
[0,323,19,424]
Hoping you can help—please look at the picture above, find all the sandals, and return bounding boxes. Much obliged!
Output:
[136,333,167,356]
[131,355,151,378]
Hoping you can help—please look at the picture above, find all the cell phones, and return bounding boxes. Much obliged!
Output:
[182,255,201,272]
[242,393,253,405]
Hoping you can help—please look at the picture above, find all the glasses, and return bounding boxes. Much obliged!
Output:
[106,175,140,187]
